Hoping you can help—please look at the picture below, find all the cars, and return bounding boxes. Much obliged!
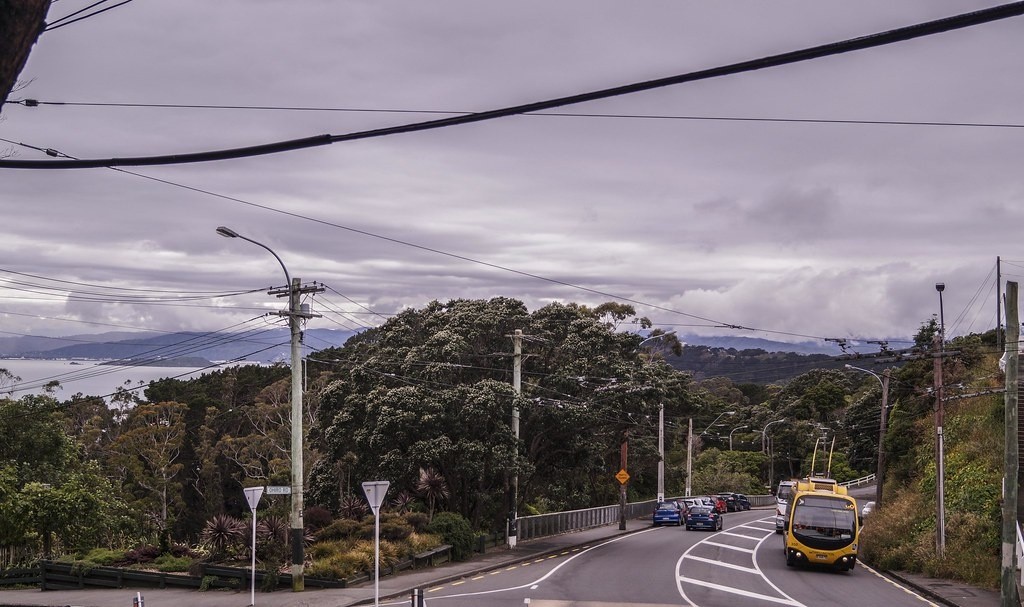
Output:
[685,505,724,534]
[653,490,752,527]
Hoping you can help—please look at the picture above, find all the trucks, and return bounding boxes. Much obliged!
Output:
[773,474,798,534]
[781,477,863,576]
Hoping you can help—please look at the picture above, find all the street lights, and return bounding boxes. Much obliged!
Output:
[846,362,891,510]
[218,227,292,314]
